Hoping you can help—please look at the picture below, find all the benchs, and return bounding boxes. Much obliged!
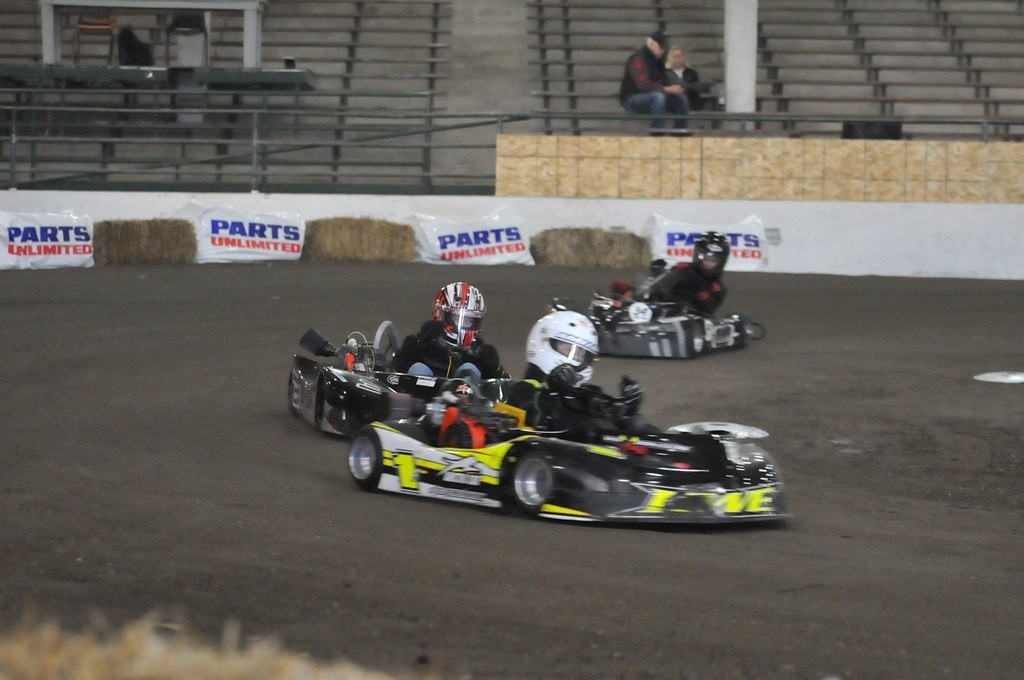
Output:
[522,0,1024,140]
[0,0,455,192]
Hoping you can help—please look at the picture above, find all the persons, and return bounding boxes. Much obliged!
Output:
[619,31,702,135]
[390,282,501,380]
[612,230,731,314]
[507,311,642,441]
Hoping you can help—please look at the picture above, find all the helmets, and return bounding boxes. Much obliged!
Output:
[432,281,486,350]
[526,311,598,388]
[693,230,729,279]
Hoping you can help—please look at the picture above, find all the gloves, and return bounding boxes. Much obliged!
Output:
[541,364,585,400]
[618,376,643,407]
[476,345,499,376]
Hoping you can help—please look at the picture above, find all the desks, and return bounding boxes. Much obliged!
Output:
[41,0,268,67]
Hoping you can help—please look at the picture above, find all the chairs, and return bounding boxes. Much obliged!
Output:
[165,10,209,69]
[72,8,117,65]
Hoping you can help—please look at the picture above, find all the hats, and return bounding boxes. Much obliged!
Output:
[650,30,670,49]
